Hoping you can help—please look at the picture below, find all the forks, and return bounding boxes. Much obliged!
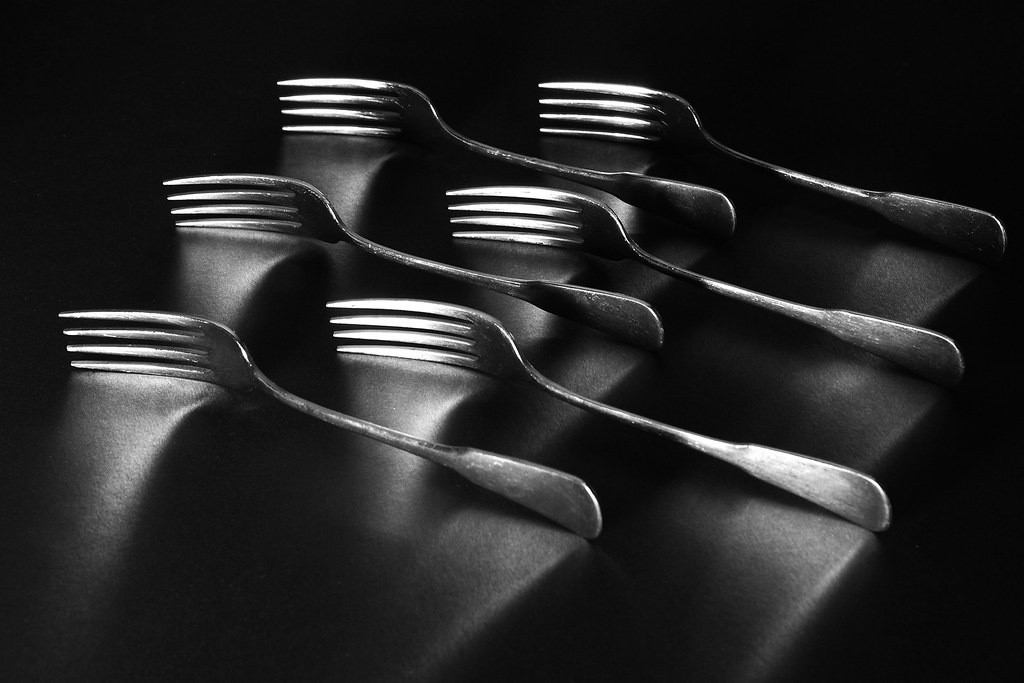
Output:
[278,78,735,236]
[446,185,965,386]
[60,311,604,540]
[325,298,892,533]
[163,176,664,349]
[539,83,1007,260]
